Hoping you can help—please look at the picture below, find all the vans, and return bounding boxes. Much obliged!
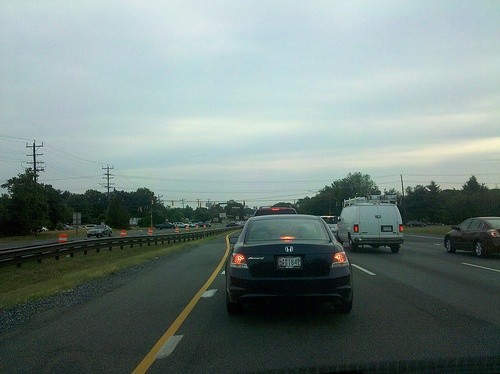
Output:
[336,194,405,254]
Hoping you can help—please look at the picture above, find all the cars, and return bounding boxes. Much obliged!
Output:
[224,213,353,316]
[226,205,341,241]
[86,224,113,238]
[28,222,97,234]
[406,220,427,228]
[155,220,212,230]
[443,216,500,257]
